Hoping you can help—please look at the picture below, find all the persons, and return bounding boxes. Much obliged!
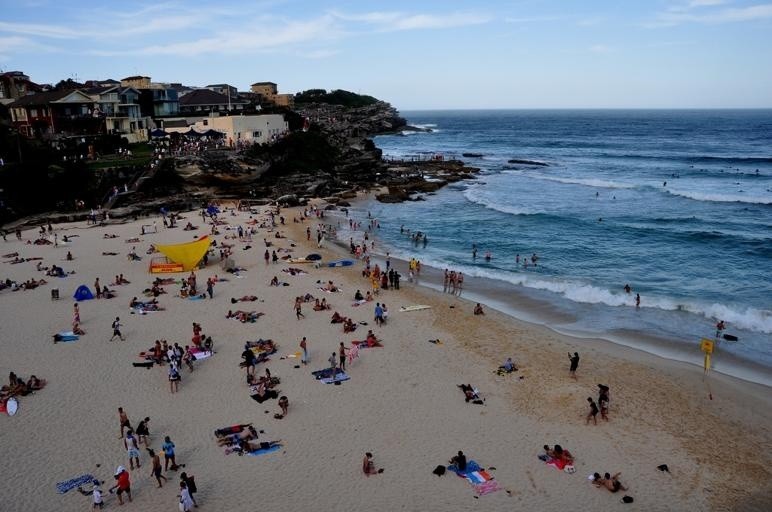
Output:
[592,472,629,493]
[471,237,538,268]
[363,452,377,474]
[429,339,443,345]
[214,422,284,454]
[456,383,479,403]
[474,302,485,315]
[93,466,134,510]
[599,218,602,222]
[544,444,575,461]
[118,407,150,471]
[505,357,518,372]
[449,451,467,471]
[1,199,428,419]
[716,321,726,337]
[63,102,456,197]
[149,435,198,510]
[568,352,579,377]
[587,384,609,425]
[444,268,464,289]
[623,284,640,306]
